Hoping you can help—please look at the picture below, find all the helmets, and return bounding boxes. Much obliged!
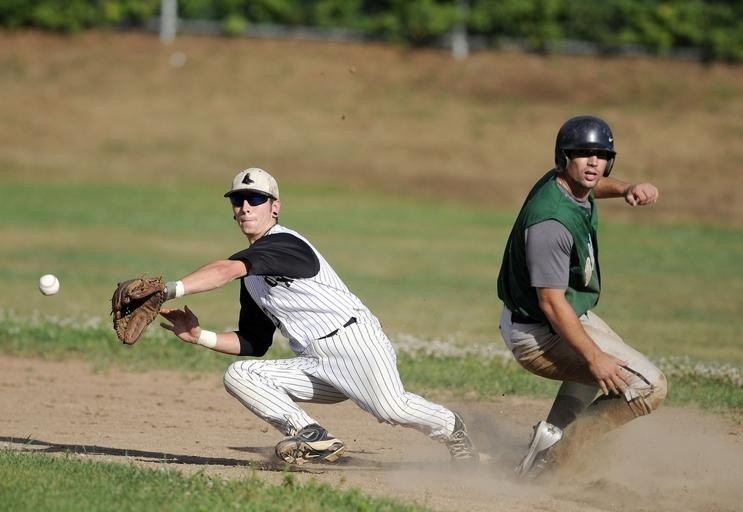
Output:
[554,115,616,177]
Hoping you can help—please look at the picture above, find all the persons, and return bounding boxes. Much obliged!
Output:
[110,168,480,478]
[496,116,668,488]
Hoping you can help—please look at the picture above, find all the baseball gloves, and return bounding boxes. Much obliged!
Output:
[109,277,168,345]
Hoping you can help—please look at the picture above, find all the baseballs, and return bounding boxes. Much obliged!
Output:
[40,274,60,296]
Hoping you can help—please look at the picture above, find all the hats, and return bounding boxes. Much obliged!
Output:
[223,167,279,200]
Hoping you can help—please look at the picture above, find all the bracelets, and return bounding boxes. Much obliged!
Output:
[165,280,185,303]
[196,329,217,349]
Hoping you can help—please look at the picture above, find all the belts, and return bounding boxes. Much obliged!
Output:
[318,317,355,339]
[510,311,542,324]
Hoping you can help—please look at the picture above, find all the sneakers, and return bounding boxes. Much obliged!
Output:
[438,410,479,467]
[513,420,564,484]
[275,423,344,465]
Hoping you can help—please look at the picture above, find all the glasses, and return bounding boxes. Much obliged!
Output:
[230,192,275,207]
